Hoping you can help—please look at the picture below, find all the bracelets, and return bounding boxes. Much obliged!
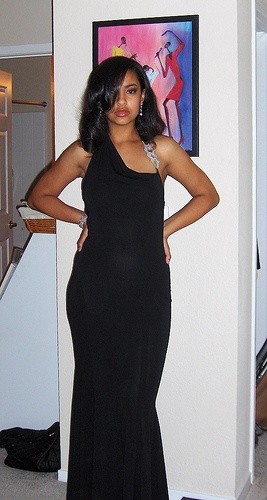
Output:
[76,213,90,230]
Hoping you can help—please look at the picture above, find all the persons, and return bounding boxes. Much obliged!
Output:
[24,57,220,500]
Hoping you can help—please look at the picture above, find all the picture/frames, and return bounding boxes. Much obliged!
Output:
[92,14,199,157]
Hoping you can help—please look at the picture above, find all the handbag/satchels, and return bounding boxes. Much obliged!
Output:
[0,422,60,472]
